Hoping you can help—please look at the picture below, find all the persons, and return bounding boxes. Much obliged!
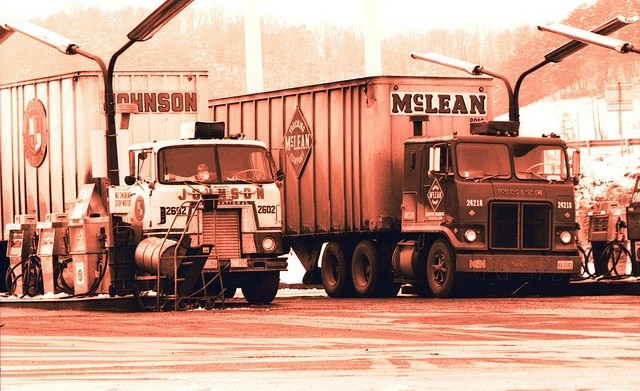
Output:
[196,163,210,181]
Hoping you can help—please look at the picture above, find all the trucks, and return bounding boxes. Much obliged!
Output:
[2,71,288,311]
[209,78,582,300]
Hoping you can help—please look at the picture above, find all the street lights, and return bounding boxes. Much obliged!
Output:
[5,0,195,187]
[412,14,640,123]
